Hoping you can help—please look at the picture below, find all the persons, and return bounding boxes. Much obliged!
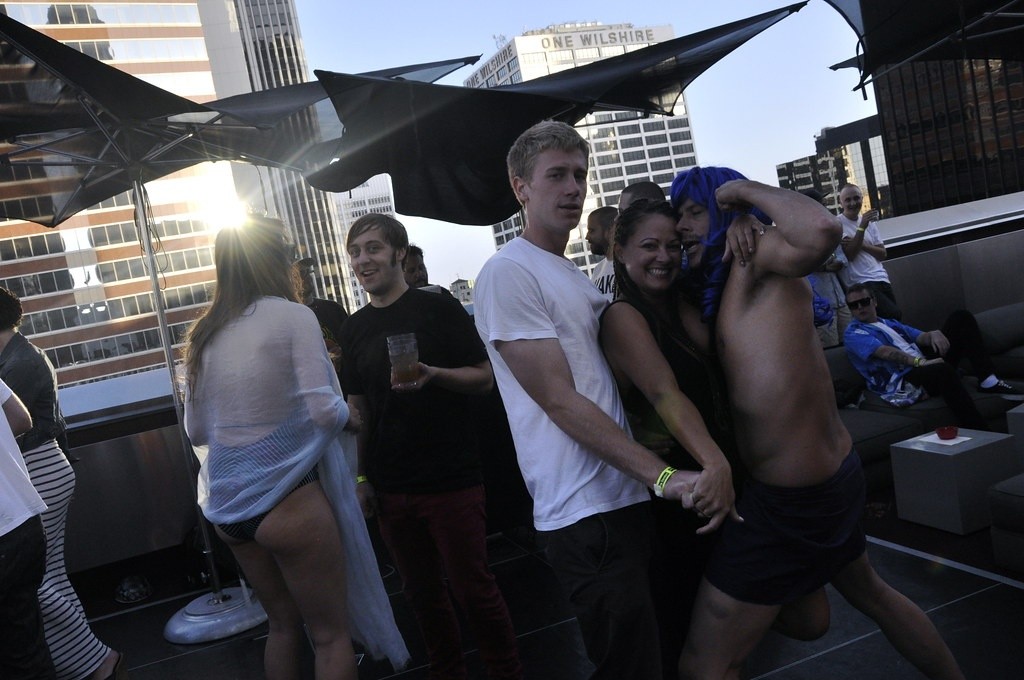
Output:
[0,118,1024,680]
[473,119,702,680]
[183,217,359,680]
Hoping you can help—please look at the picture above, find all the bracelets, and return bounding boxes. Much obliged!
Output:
[857,227,865,232]
[653,466,676,498]
[913,356,921,368]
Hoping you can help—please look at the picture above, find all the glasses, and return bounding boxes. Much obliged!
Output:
[847,296,873,311]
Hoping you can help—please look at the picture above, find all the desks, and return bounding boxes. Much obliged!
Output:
[890,429,1017,536]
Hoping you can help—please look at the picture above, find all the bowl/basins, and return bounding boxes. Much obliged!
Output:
[936,425,959,440]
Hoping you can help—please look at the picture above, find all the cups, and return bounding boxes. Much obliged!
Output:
[388,331,420,387]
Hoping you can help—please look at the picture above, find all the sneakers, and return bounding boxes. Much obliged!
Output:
[976,378,1024,400]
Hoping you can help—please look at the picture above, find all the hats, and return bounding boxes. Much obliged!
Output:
[805,188,830,203]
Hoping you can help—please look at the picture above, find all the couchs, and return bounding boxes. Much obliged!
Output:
[823,301,1024,487]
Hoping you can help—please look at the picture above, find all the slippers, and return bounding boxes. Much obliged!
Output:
[93,651,122,679]
